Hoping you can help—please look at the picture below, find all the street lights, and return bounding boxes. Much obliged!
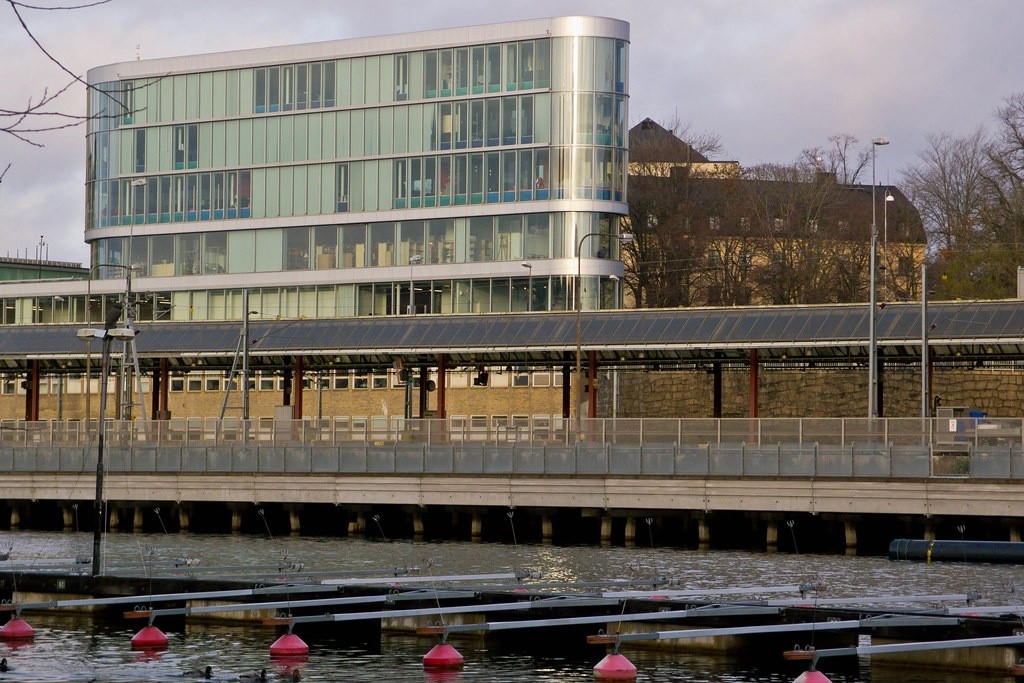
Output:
[409,255,423,432]
[521,262,533,312]
[76,328,136,576]
[610,275,620,444]
[54,296,65,422]
[884,190,895,302]
[576,233,633,441]
[869,137,890,434]
[85,264,142,450]
[217,310,258,434]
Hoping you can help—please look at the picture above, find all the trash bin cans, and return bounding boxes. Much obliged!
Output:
[931,406,970,478]
[969,411,988,429]
